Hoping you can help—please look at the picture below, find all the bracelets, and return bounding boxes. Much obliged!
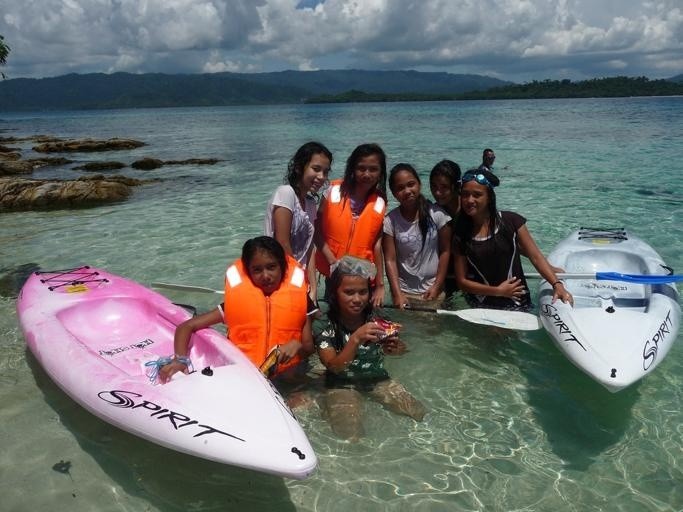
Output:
[146,350,196,375]
[553,281,562,289]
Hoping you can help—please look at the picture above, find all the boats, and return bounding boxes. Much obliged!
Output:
[536,226,681,394]
[15,265,317,479]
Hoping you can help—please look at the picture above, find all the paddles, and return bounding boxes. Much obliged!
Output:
[316,298,540,330]
[151,282,226,294]
[524,272,683,283]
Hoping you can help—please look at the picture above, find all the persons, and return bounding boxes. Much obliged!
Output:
[263,141,332,320]
[379,163,453,337]
[428,159,567,305]
[156,236,316,409]
[477,148,500,177]
[311,255,427,444]
[315,143,408,355]
[451,169,574,313]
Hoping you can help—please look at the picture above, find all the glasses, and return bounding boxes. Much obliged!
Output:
[462,173,494,189]
[487,157,495,160]
